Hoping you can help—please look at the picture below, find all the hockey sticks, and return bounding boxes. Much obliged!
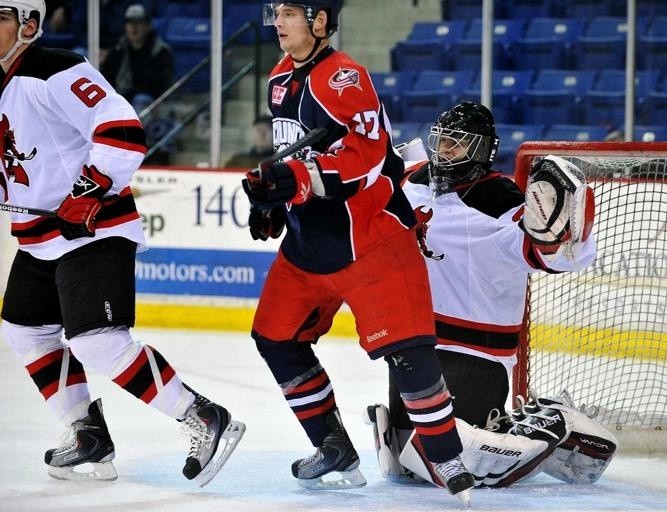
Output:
[258,128,327,187]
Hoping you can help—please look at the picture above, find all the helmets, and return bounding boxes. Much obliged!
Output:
[428,100,499,196]
[0,0,46,37]
[262,0,343,30]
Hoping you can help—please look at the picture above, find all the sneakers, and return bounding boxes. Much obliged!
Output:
[430,455,474,495]
[292,427,360,479]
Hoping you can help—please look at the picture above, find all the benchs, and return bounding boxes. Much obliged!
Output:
[36,1,277,163]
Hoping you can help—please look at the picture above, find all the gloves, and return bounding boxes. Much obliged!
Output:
[56,164,113,240]
[246,158,313,209]
[242,168,287,241]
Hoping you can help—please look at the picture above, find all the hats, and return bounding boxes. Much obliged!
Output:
[125,4,151,23]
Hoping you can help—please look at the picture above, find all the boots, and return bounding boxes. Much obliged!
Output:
[177,381,232,480]
[44,398,115,467]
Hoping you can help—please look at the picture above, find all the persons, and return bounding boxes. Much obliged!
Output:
[370,100,597,491]
[241,0,476,497]
[0,0,230,478]
[98,4,179,106]
